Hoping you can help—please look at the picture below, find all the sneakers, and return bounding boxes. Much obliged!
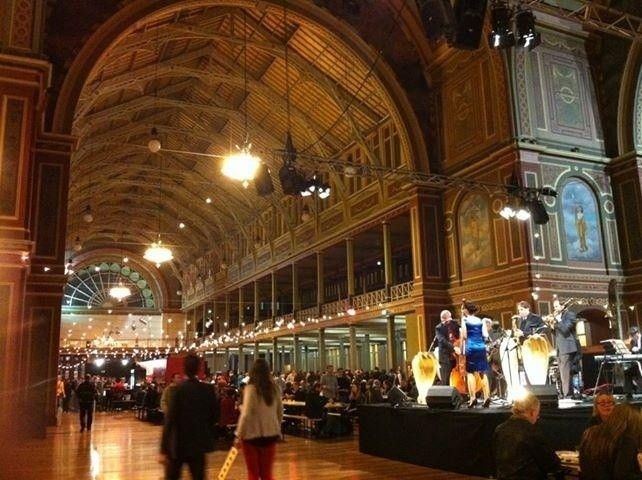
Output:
[80,426,91,432]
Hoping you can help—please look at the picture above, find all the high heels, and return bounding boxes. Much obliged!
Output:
[468,399,478,409]
[482,398,491,409]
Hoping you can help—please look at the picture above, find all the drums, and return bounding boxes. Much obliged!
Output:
[550,366,561,382]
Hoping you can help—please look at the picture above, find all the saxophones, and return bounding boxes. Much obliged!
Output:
[511,315,523,361]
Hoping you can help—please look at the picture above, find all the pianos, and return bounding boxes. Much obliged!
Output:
[594,354,642,363]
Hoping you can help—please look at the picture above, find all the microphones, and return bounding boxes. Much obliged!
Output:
[512,316,521,319]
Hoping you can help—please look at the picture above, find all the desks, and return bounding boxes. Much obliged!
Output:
[282,399,349,438]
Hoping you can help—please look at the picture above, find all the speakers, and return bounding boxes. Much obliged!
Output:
[387,385,410,406]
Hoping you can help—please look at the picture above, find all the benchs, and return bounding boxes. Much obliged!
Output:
[280,413,355,440]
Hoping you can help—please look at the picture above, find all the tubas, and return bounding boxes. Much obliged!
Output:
[541,297,574,331]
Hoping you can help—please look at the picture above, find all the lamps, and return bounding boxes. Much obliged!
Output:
[176,235,261,295]
[82,134,95,223]
[389,385,407,405]
[123,255,129,263]
[148,128,162,153]
[256,143,332,221]
[74,225,81,252]
[501,194,549,225]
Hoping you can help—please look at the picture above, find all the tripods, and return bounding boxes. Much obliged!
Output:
[497,320,516,410]
[483,365,507,403]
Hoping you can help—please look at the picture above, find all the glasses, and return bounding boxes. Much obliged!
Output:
[597,401,616,407]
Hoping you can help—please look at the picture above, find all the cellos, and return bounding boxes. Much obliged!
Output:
[449,298,482,396]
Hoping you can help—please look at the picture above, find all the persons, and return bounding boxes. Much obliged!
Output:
[158,355,223,480]
[56,372,181,433]
[198,360,419,433]
[584,390,617,429]
[233,358,285,480]
[580,404,642,479]
[490,391,561,480]
[435,296,580,408]
[573,196,588,254]
[622,326,642,399]
